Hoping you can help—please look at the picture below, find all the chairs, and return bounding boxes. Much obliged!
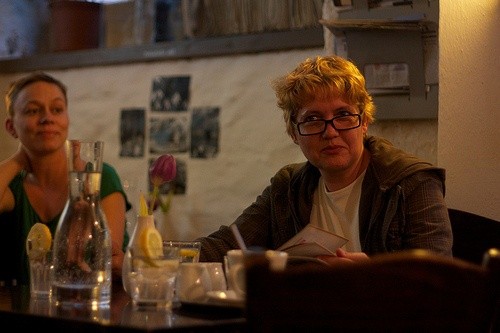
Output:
[244,248,500,332]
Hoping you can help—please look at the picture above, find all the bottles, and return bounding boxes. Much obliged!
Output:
[52,140,113,306]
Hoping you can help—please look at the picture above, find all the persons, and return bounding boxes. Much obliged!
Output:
[190,55,453,265]
[0,72,133,273]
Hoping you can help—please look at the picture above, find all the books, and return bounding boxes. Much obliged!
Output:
[276,224,350,265]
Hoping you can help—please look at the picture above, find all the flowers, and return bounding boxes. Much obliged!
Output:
[137,153,176,215]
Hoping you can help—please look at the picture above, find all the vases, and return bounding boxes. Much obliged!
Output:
[121,216,155,304]
[49,0,101,51]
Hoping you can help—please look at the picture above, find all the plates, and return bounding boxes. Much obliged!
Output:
[207,290,246,308]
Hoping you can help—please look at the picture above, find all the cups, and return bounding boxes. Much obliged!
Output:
[129,247,181,310]
[175,263,229,303]
[225,249,288,298]
[26,238,53,298]
[163,241,202,302]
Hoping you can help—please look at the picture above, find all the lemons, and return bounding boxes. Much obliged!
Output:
[142,229,163,259]
[26,223,51,256]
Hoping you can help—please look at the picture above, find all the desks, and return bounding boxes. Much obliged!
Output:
[0,269,246,333]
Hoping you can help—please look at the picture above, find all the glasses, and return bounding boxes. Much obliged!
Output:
[290,107,365,137]
